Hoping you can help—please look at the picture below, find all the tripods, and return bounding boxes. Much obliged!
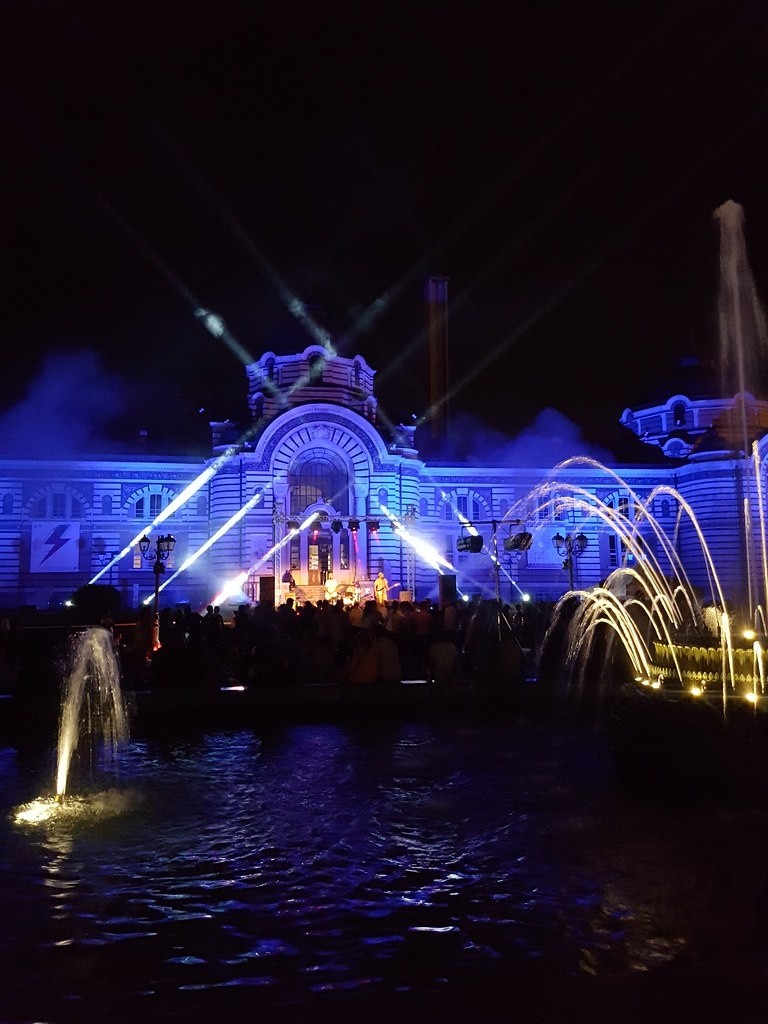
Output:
[487,522,532,665]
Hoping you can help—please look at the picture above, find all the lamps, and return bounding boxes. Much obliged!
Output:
[390,520,402,533]
[287,522,300,534]
[310,522,322,534]
[366,522,381,533]
[331,522,344,535]
[347,520,361,534]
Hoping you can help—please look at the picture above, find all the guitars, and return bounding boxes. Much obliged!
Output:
[373,581,401,600]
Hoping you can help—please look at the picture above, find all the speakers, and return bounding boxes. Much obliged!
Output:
[260,577,275,608]
[438,575,456,610]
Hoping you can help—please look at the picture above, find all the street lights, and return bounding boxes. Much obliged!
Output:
[139,530,175,655]
[551,532,588,598]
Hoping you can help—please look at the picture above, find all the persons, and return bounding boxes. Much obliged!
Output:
[324,573,339,603]
[99,590,578,682]
[374,572,389,603]
[282,570,297,591]
